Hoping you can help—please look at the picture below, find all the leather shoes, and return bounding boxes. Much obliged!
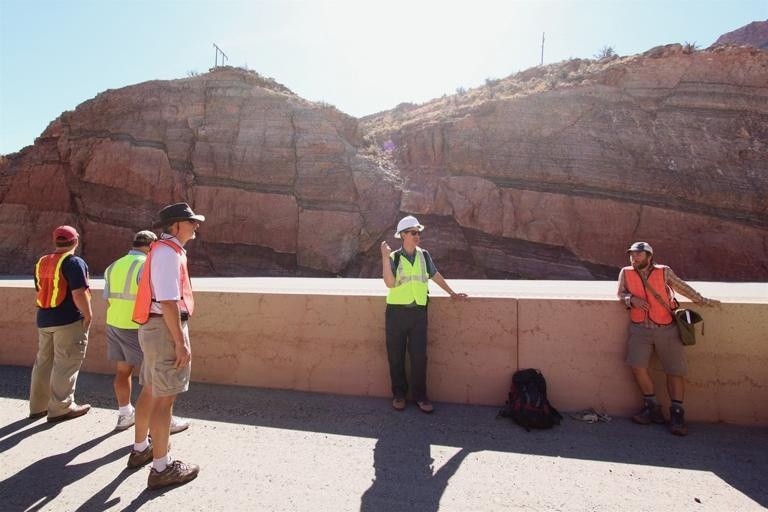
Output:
[29,409,47,419]
[47,403,91,423]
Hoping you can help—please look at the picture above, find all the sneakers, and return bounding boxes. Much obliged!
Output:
[149,415,189,437]
[127,438,171,469]
[392,396,406,411]
[146,460,199,491]
[415,398,434,412]
[669,405,687,436]
[631,400,664,425]
[115,407,134,431]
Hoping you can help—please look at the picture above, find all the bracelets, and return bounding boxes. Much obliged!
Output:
[449,291,454,294]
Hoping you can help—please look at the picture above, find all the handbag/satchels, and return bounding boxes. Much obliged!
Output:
[673,308,703,346]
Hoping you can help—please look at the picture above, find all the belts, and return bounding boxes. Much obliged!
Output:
[149,312,189,322]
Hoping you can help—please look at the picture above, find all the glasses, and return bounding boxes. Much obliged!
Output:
[404,231,420,236]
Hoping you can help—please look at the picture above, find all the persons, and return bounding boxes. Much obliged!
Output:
[102,230,189,435]
[127,202,205,490]
[616,241,721,436]
[380,215,468,413]
[29,226,93,422]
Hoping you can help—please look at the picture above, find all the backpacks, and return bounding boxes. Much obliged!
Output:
[498,368,563,430]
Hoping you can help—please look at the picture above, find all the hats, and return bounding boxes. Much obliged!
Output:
[393,215,424,239]
[623,242,652,256]
[132,230,158,243]
[153,201,205,229]
[53,225,80,243]
